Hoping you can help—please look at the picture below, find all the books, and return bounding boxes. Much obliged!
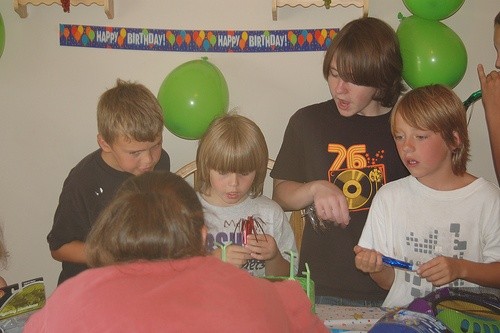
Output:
[1,278,45,317]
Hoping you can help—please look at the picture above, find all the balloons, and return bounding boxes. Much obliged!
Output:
[402,0,465,19]
[395,12,468,90]
[157,56,230,140]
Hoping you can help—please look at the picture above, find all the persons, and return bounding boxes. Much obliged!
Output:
[194,114,299,278]
[354,84,500,310]
[270,16,411,307]
[47,79,170,285]
[477,10,500,187]
[23,171,329,333]
